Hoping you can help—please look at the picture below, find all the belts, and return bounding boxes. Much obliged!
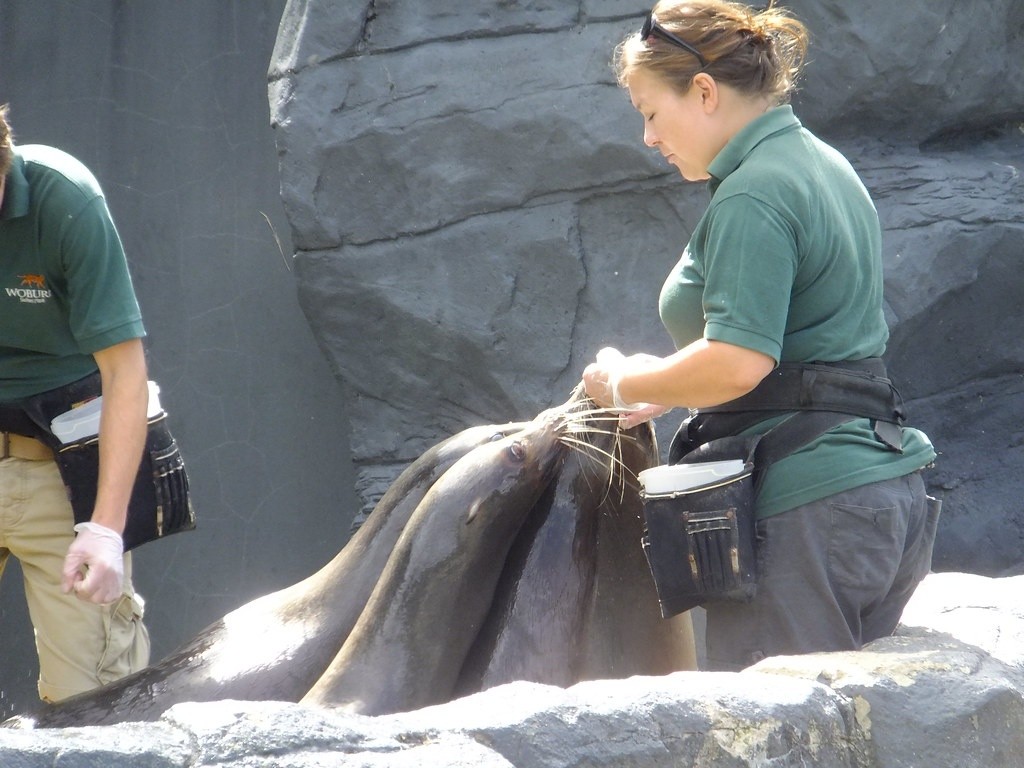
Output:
[0,430,56,461]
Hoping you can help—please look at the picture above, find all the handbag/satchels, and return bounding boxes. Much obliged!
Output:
[1,361,198,553]
[638,412,757,616]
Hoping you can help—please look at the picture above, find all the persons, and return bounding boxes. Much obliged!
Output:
[581,1,944,675]
[0,100,153,702]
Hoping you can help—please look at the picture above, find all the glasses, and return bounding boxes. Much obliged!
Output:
[640,9,708,68]
